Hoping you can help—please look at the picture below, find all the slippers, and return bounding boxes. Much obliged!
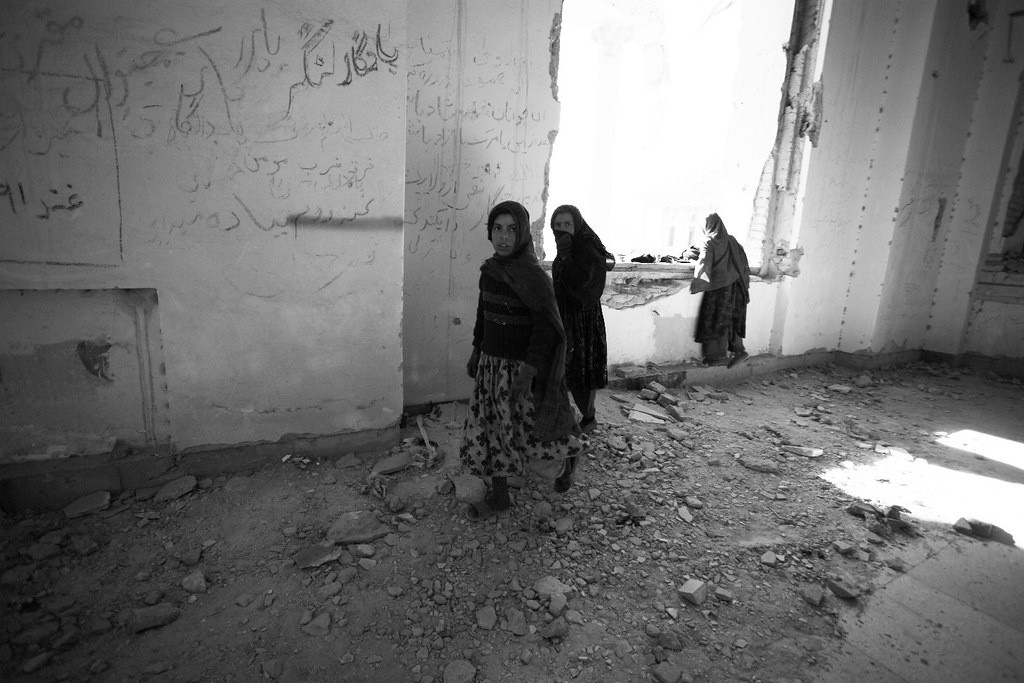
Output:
[554,453,579,493]
[466,501,516,521]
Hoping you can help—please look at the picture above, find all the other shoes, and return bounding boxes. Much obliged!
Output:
[702,356,730,365]
[727,351,749,369]
[581,419,597,433]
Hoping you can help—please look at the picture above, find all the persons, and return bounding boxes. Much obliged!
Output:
[460,201,590,522]
[550,203,615,433]
[689,213,750,367]
